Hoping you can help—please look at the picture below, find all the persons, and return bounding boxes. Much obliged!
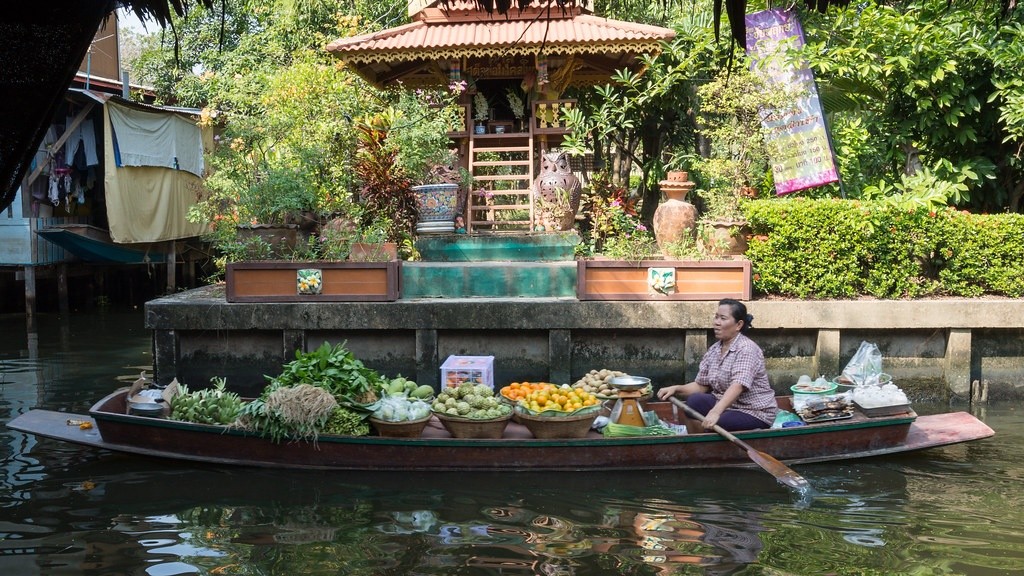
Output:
[656,298,778,433]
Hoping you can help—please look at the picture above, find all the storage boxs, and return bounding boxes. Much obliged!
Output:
[125,370,180,418]
[852,401,912,416]
[439,354,495,393]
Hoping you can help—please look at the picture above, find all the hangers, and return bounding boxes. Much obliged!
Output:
[54,151,71,177]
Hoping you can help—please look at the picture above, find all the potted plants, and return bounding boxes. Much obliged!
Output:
[473,92,489,134]
[660,150,698,182]
[690,76,800,256]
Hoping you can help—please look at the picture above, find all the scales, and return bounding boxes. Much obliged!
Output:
[605,375,652,427]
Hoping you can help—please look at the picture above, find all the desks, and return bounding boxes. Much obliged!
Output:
[488,122,514,134]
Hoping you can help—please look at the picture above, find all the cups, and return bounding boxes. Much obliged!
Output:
[495,126,505,134]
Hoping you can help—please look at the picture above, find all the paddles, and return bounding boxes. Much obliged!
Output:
[669,395,812,488]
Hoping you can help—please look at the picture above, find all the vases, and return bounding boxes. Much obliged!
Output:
[696,220,751,254]
[411,183,460,221]
[495,126,505,134]
[575,254,753,300]
[652,188,699,254]
[226,260,404,302]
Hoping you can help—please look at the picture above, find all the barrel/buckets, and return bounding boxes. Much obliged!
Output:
[129,402,165,418]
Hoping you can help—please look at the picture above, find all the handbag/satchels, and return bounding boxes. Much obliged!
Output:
[842,339,885,386]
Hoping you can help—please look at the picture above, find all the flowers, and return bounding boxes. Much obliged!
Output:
[296,269,323,295]
[220,223,393,262]
[572,197,763,270]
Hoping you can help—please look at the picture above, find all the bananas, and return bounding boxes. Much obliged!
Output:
[168,389,246,424]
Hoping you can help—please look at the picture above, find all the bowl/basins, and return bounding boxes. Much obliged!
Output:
[790,383,837,411]
[834,372,892,393]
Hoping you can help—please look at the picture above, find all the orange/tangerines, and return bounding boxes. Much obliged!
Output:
[518,384,596,412]
[502,381,556,400]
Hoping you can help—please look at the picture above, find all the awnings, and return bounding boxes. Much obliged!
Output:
[29,87,221,186]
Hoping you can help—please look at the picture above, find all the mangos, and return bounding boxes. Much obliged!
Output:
[385,376,433,399]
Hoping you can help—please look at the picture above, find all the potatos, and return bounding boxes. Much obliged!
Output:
[574,370,648,396]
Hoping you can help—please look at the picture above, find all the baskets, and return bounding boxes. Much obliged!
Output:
[833,371,893,392]
[366,378,655,439]
[790,382,838,396]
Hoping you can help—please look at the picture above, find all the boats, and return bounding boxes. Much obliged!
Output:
[3,386,998,474]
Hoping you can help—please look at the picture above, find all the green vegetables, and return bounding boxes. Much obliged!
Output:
[603,423,673,437]
[238,338,392,436]
